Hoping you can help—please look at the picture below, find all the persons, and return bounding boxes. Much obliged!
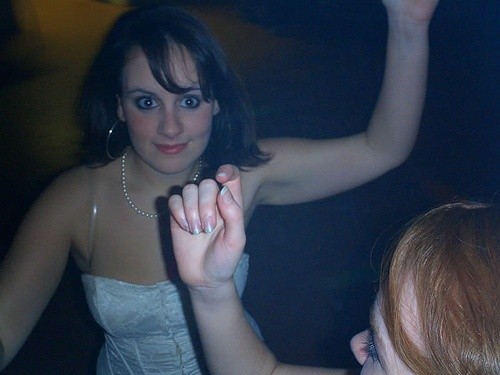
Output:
[0,0,436,375]
[166,164,499,375]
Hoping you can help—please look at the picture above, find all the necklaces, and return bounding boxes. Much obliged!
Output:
[122,151,203,219]
